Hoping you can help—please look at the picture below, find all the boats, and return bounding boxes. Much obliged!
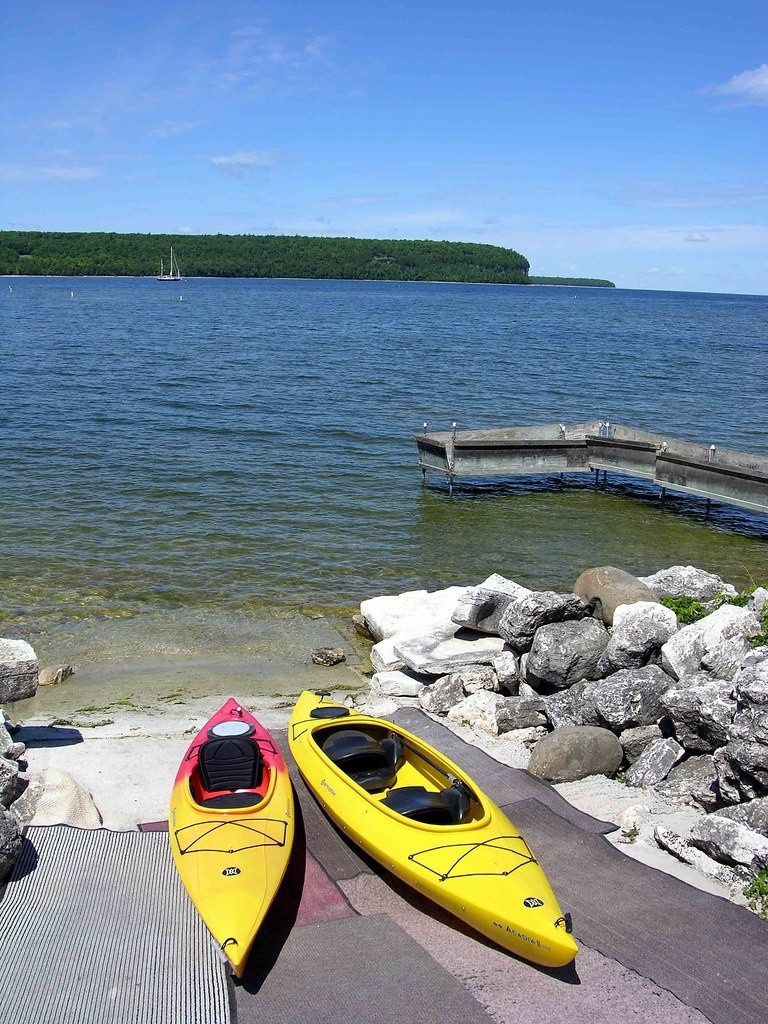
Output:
[168,696,295,980]
[286,689,579,968]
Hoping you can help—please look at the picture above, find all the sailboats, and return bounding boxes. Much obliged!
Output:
[157,246,181,281]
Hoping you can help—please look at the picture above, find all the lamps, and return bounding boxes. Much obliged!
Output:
[708,444,717,462]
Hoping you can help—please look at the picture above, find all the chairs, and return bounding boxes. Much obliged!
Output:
[323,736,403,790]
[381,785,470,825]
[197,736,265,810]
